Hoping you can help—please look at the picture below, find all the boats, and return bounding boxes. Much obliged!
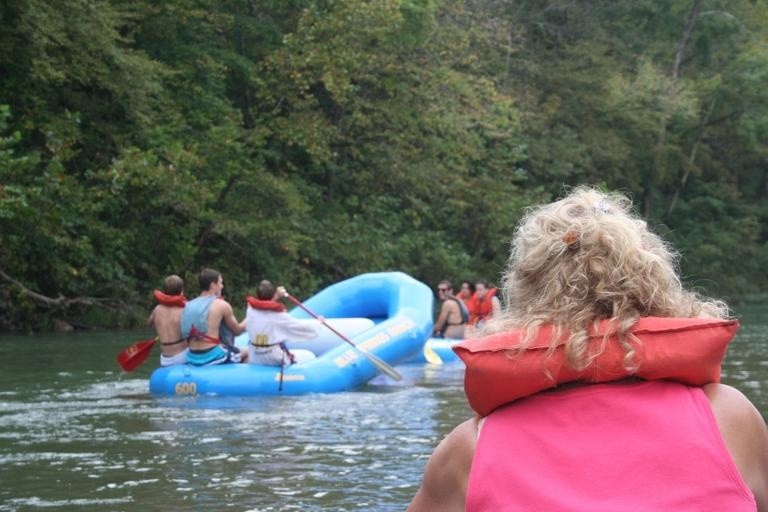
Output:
[402,337,464,366]
[144,266,434,394]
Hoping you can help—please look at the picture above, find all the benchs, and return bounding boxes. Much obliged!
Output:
[282,317,375,357]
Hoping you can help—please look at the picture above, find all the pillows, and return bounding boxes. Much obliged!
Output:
[152,288,186,307]
[451,315,740,418]
[246,295,286,312]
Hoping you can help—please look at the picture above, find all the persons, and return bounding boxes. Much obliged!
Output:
[244,280,326,368]
[146,274,190,367]
[406,184,767,512]
[431,280,470,340]
[180,267,249,369]
[471,280,501,322]
[456,280,477,326]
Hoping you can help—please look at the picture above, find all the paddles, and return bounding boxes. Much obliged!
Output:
[283,293,403,381]
[118,336,159,373]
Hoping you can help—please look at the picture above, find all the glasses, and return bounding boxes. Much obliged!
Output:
[437,288,451,292]
